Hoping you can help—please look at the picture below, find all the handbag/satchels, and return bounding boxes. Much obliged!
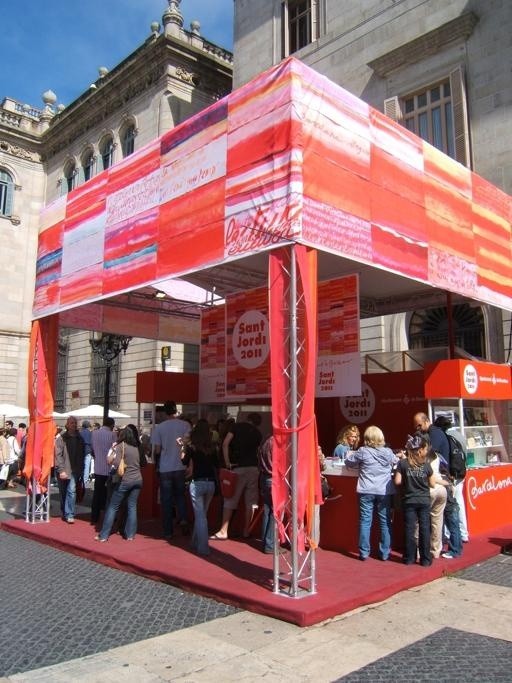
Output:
[320,472,342,503]
[218,463,239,498]
[116,441,127,476]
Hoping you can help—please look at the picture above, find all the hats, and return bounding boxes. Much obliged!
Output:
[164,401,177,414]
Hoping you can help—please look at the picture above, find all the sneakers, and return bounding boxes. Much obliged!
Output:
[442,551,454,559]
[94,536,108,542]
[67,518,74,523]
[265,547,288,554]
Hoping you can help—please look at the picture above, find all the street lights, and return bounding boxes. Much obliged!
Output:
[89,332,132,419]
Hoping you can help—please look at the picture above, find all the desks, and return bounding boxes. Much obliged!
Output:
[112,459,425,550]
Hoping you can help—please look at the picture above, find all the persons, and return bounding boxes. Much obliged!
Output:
[4,420,13,432]
[79,421,92,485]
[257,434,288,554]
[394,431,435,566]
[16,423,26,447]
[9,428,22,488]
[94,424,142,541]
[150,400,192,539]
[176,419,216,556]
[208,412,263,540]
[20,426,26,489]
[54,417,85,524]
[413,412,464,559]
[0,427,11,490]
[332,425,360,459]
[423,433,450,559]
[344,426,399,561]
[310,445,330,551]
[90,417,119,525]
[433,416,469,543]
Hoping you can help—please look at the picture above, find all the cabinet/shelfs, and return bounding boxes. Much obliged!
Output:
[426,361,512,539]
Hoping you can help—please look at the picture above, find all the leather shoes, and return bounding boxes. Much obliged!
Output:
[182,525,190,535]
[359,556,366,561]
[155,529,173,539]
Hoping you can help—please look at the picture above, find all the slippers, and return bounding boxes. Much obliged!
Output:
[209,533,227,539]
